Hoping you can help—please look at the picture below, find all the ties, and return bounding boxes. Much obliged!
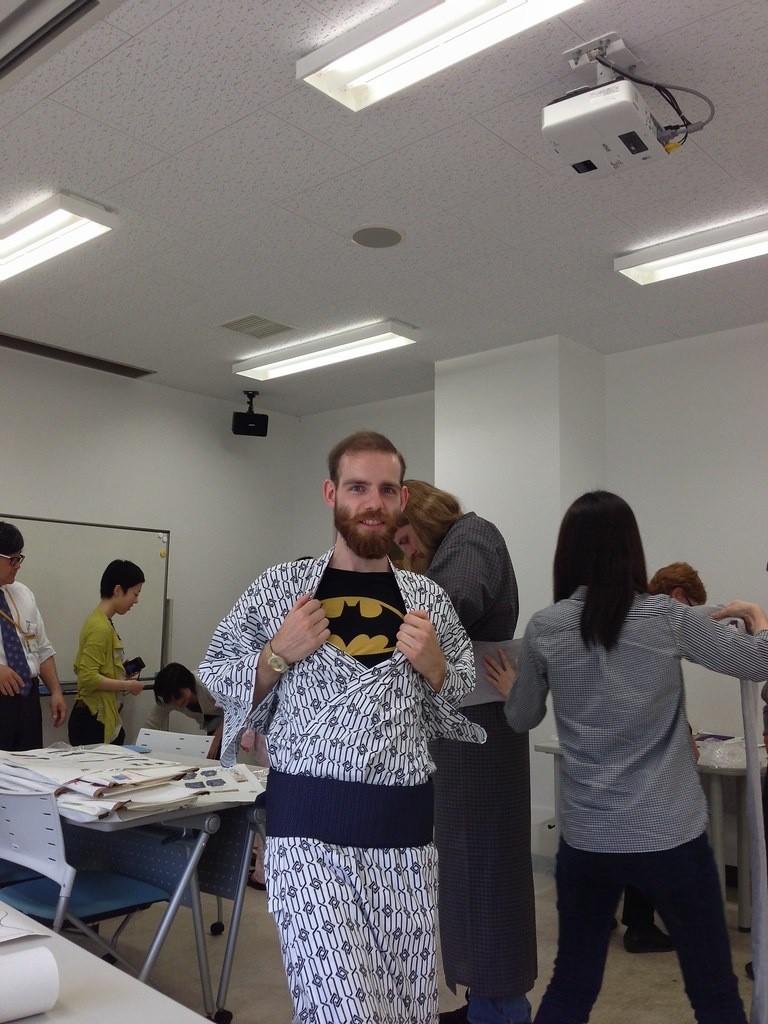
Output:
[0,588,33,698]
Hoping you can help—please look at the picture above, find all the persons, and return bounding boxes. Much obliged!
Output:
[143,663,266,891]
[0,521,68,751]
[481,491,768,1024]
[393,479,537,1024]
[197,430,488,1024]
[68,560,145,745]
[609,563,707,955]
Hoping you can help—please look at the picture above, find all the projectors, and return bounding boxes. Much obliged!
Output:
[542,80,670,184]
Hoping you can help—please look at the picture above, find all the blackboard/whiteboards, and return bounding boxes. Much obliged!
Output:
[0,513,171,696]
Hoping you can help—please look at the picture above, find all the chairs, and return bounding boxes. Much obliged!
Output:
[0,789,170,988]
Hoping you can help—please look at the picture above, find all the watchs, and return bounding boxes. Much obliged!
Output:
[265,640,291,674]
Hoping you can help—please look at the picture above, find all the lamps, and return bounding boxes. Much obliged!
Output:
[232,390,269,438]
[1,193,117,282]
[295,0,587,115]
[614,213,768,286]
[231,321,419,381]
[0,0,126,97]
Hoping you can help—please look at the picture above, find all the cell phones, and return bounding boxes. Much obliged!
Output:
[123,656,146,675]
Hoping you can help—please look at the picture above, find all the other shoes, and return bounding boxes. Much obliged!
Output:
[623,924,677,954]
[610,917,618,930]
[745,961,755,981]
[438,986,533,1024]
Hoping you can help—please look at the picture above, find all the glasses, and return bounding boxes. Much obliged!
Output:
[0,554,26,566]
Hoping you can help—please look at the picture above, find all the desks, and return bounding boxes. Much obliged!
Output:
[533,740,768,931]
[0,900,219,1024]
[55,741,269,1024]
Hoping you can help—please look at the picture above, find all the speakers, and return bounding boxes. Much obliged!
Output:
[231,411,269,437]
